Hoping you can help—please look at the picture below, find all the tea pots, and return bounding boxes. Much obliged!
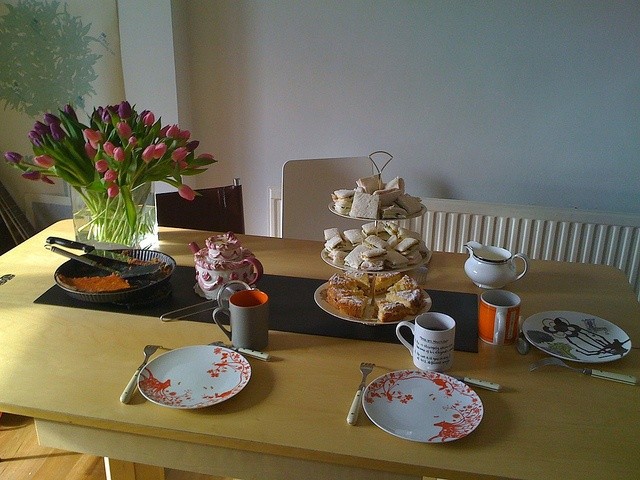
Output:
[463,240,528,290]
[189,231,263,298]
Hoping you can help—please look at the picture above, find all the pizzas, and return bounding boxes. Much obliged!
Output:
[55,249,173,293]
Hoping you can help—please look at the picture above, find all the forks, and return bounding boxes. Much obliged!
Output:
[346,361,375,426]
[529,356,637,386]
[120,345,163,404]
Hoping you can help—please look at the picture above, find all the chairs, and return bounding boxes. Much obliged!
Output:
[158,178,245,235]
[279,156,375,242]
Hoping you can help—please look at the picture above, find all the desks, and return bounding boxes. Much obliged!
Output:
[0,217,639,479]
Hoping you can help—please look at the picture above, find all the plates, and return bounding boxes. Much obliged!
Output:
[320,247,432,274]
[194,282,257,300]
[314,282,433,325]
[362,369,484,444]
[327,201,427,222]
[136,345,252,409]
[522,310,632,363]
[53,249,176,303]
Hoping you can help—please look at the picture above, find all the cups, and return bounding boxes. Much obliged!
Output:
[478,290,521,347]
[212,289,269,351]
[396,311,456,372]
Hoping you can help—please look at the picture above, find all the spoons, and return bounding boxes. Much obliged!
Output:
[450,375,505,394]
[208,340,272,363]
[516,315,530,355]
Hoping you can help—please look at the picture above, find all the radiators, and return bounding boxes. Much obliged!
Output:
[266,187,640,303]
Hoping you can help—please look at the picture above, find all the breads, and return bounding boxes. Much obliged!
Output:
[323,221,429,271]
[331,174,424,220]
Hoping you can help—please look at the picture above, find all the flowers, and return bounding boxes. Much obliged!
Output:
[4,100,218,260]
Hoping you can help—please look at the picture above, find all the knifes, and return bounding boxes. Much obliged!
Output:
[46,237,143,265]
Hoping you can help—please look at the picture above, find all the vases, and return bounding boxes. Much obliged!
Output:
[70,181,161,252]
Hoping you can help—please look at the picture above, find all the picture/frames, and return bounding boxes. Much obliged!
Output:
[24,193,71,231]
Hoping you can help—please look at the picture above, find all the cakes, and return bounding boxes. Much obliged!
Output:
[326,271,424,324]
